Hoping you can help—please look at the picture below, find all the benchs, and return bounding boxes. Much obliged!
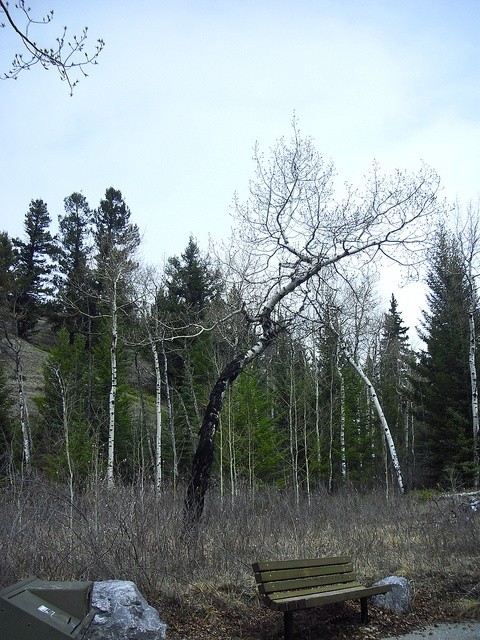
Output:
[251,555,392,638]
